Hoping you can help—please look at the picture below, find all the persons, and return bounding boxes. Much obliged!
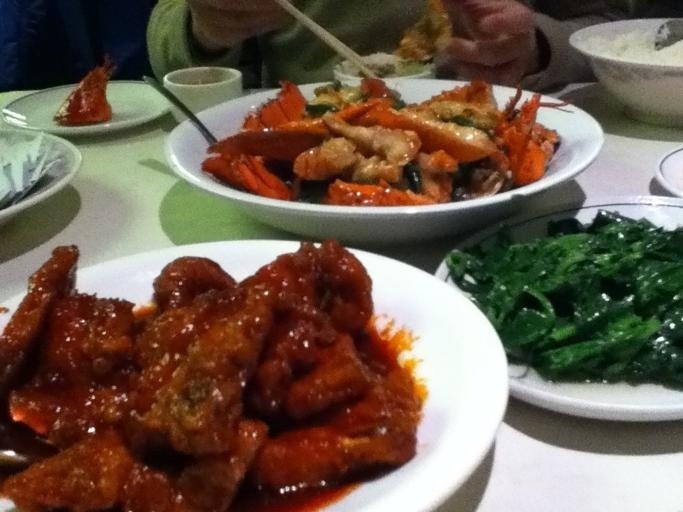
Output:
[145,0,631,95]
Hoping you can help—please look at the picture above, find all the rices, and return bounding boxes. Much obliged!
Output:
[343,51,398,77]
[581,24,683,66]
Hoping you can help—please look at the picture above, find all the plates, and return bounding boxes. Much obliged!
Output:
[651,146,683,198]
[430,201,683,424]
[1,79,173,135]
[0,129,82,215]
[0,238,512,512]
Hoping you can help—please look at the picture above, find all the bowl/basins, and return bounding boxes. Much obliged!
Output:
[566,15,683,133]
[332,53,434,86]
[165,78,605,246]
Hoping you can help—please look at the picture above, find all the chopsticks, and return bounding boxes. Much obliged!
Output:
[278,0,376,78]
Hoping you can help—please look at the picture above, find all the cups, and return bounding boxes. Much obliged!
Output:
[162,65,243,120]
